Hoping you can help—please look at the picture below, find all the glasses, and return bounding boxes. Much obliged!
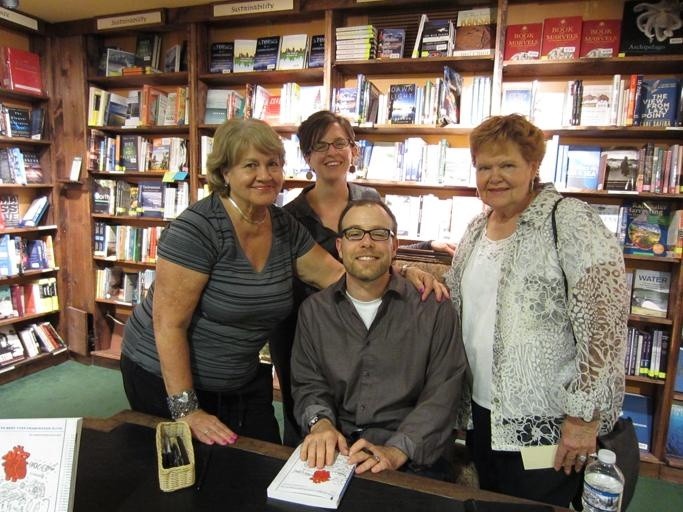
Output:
[340,226,395,240]
[307,138,353,152]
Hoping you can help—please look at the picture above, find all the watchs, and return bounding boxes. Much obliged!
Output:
[303,412,332,430]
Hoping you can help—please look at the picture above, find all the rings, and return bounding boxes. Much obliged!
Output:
[369,453,383,462]
[577,455,588,461]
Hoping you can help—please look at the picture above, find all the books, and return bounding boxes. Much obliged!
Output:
[1,2,683,262]
[257,340,285,385]
[617,259,682,462]
[266,442,360,510]
[1,417,82,511]
[1,222,169,374]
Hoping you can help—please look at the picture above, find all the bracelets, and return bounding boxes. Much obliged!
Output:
[396,257,410,279]
[164,390,200,420]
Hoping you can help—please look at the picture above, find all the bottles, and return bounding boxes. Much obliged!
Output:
[580,449,625,512]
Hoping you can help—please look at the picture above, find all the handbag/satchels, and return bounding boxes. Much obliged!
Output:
[569,417,639,512]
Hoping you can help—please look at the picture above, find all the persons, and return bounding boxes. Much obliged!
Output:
[288,197,470,484]
[275,110,387,451]
[440,111,632,507]
[118,116,453,447]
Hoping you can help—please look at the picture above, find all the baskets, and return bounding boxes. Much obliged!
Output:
[156,421,196,493]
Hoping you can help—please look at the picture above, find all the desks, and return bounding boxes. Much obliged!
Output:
[73,409,577,512]
